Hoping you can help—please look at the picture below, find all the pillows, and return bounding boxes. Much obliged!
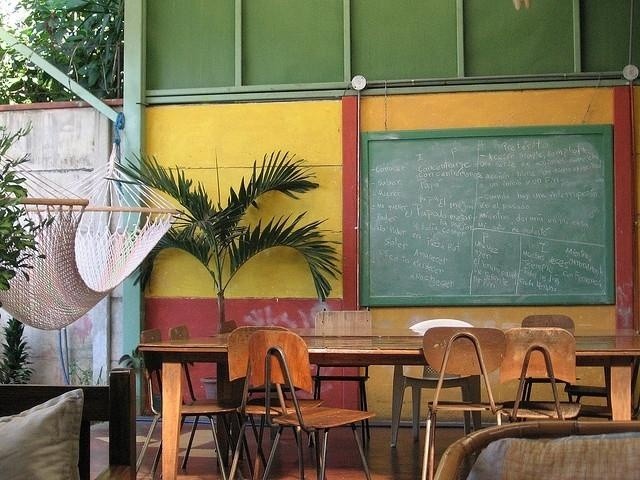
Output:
[0,390,85,480]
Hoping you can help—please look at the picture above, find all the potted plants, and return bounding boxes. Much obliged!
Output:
[105,150,346,424]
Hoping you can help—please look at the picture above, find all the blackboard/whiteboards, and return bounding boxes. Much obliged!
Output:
[359,126,612,305]
